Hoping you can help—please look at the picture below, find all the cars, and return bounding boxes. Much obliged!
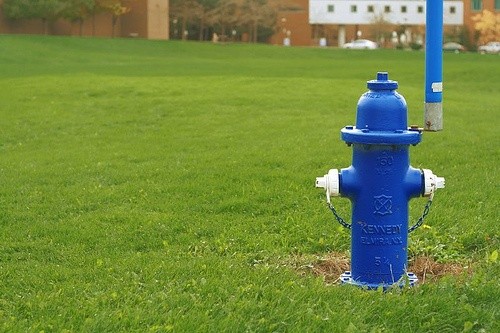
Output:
[344,39,378,49]
[443,41,465,54]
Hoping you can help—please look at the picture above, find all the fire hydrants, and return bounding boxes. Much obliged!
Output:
[314,71,447,288]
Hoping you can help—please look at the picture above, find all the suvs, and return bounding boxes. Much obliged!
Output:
[478,42,500,55]
